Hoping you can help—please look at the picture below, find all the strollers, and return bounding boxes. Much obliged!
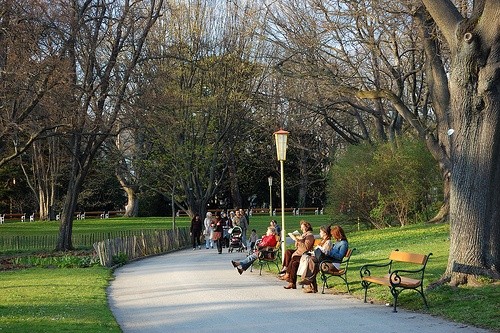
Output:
[228,225,244,253]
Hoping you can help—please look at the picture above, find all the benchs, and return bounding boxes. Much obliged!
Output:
[76,212,86,220]
[100,211,110,219]
[30,213,35,222]
[0,214,5,224]
[302,248,356,294]
[56,212,61,221]
[272,208,318,216]
[177,210,189,217]
[250,239,285,276]
[359,249,433,313]
[207,209,249,216]
[21,213,26,222]
[249,208,270,216]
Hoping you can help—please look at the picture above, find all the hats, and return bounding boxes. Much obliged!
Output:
[215,212,221,216]
[207,212,212,216]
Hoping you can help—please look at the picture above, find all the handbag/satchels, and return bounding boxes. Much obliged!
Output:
[315,248,328,261]
[203,230,207,235]
[200,231,205,243]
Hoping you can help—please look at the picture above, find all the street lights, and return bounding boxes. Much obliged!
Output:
[267,176,274,216]
[273,127,289,267]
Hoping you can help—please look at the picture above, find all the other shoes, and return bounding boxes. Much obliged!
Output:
[192,248,196,250]
[199,247,201,249]
[223,246,225,248]
[303,286,318,293]
[280,275,289,280]
[218,253,222,254]
[237,266,242,275]
[212,246,214,248]
[232,261,237,267]
[278,270,286,274]
[284,282,296,289]
[298,278,312,285]
[244,246,247,249]
[206,248,209,249]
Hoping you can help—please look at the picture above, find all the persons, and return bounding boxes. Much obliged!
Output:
[230,220,281,276]
[248,229,258,250]
[277,221,348,294]
[189,208,249,254]
